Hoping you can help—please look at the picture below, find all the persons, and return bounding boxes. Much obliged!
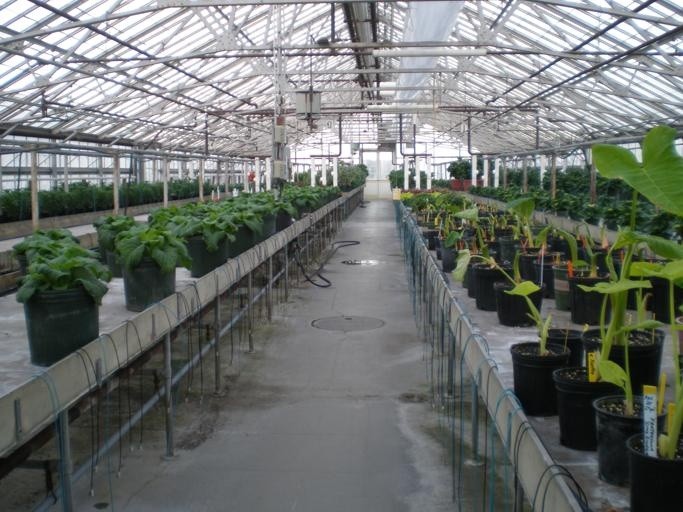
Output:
[260,171,266,192]
[248,172,255,193]
